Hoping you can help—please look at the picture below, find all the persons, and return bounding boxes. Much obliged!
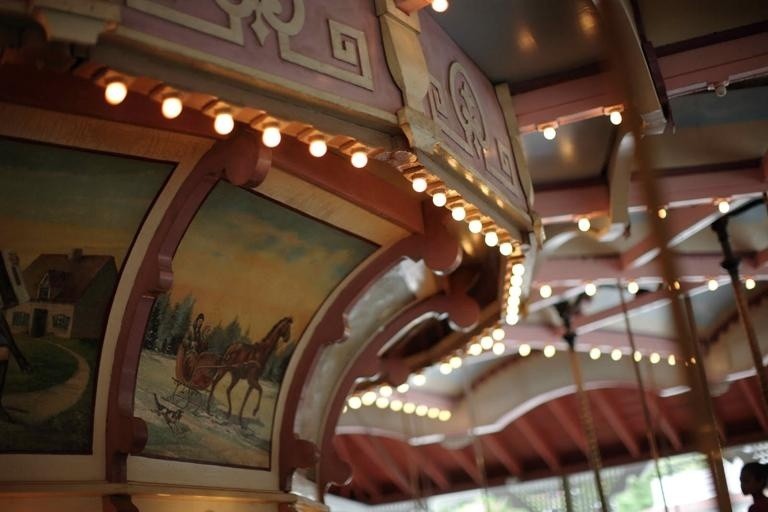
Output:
[740,462,768,512]
[182,313,204,377]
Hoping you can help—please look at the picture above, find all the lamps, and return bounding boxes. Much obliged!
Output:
[603,104,624,125]
[405,165,525,323]
[713,195,731,213]
[98,71,384,168]
[430,0,450,13]
[536,121,559,140]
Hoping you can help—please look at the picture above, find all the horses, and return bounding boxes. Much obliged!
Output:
[206,316,294,425]
